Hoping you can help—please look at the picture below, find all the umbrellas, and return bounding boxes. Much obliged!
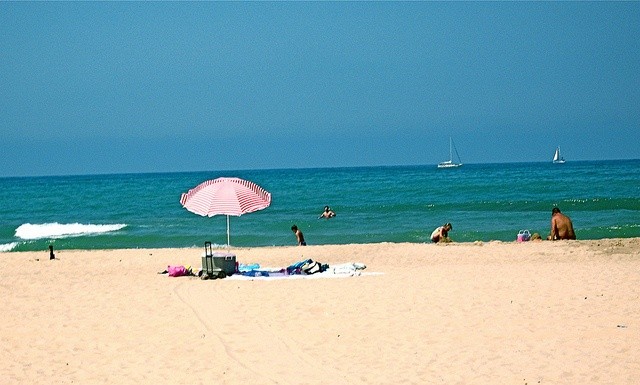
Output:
[180,177,272,246]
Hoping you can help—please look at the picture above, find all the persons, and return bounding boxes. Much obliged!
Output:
[430,223,453,243]
[291,224,306,246]
[547,207,576,240]
[317,206,336,220]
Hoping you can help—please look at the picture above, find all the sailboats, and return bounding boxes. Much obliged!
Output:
[438,136,463,168]
[552,145,566,163]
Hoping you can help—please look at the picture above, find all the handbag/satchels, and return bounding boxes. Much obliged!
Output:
[518,230,532,242]
[167,265,187,276]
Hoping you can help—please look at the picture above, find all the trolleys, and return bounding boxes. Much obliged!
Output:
[198,241,236,278]
